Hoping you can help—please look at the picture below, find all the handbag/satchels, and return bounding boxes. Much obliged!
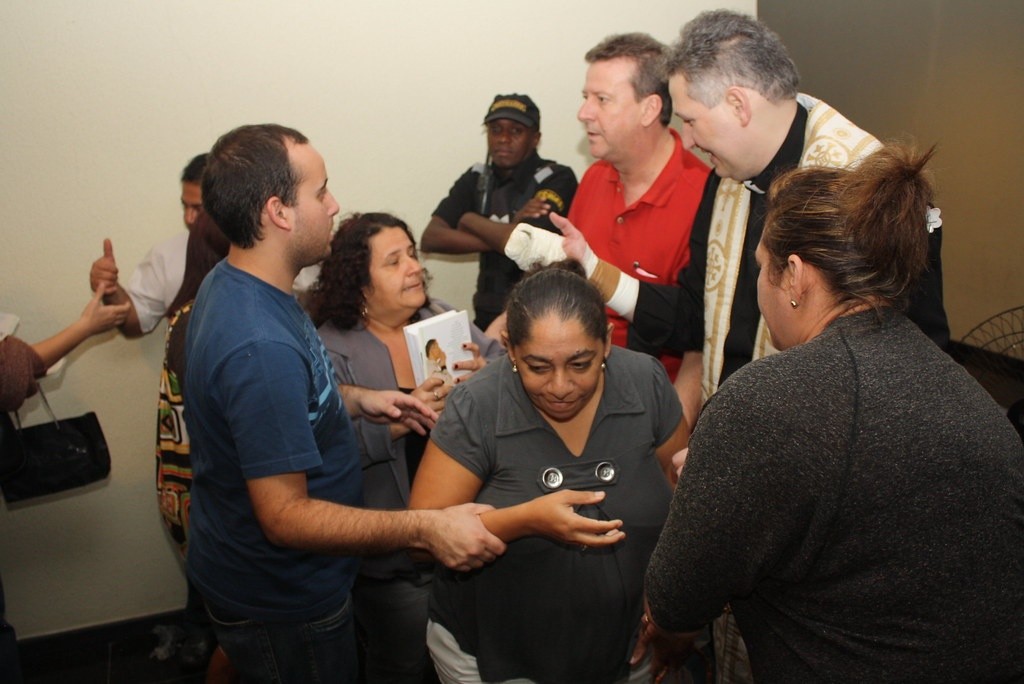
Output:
[1,382,111,504]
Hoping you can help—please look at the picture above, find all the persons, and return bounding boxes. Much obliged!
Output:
[426,339,454,386]
[0,284,131,413]
[550,10,947,480]
[565,34,710,428]
[90,125,1024,684]
[422,94,580,330]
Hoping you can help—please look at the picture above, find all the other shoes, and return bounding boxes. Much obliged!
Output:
[179,632,210,670]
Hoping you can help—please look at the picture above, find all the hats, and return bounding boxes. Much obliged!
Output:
[482,92,540,131]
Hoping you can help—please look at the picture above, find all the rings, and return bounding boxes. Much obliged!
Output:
[645,616,649,624]
[434,392,440,399]
